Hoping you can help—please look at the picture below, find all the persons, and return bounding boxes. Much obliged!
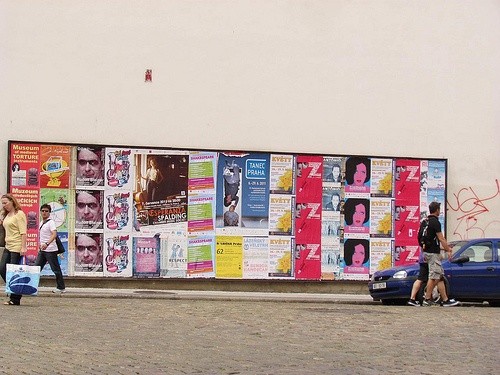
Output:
[31,204,66,293]
[296,244,300,259]
[396,166,400,180]
[296,203,301,218]
[224,159,240,212]
[77,146,104,186]
[395,206,400,221]
[297,162,303,177]
[418,201,459,307]
[0,194,28,305]
[75,233,102,272]
[327,164,342,182]
[408,212,455,307]
[140,156,163,195]
[76,190,103,229]
[326,193,340,211]
[394,246,400,261]
[225,205,239,226]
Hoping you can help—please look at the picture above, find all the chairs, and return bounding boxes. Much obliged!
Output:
[463,247,494,262]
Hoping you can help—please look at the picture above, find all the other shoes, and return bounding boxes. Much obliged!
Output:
[3,300,19,306]
[52,288,65,294]
[443,299,459,307]
[408,299,420,306]
[422,300,440,307]
[432,297,441,303]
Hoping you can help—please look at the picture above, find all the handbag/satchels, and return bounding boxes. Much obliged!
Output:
[5,255,41,296]
[0,216,5,247]
[55,235,65,254]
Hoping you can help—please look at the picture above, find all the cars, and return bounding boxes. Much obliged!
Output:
[369,238,500,306]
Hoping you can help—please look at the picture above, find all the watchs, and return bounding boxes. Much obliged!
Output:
[46,242,49,246]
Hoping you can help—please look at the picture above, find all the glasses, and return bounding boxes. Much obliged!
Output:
[40,209,49,212]
